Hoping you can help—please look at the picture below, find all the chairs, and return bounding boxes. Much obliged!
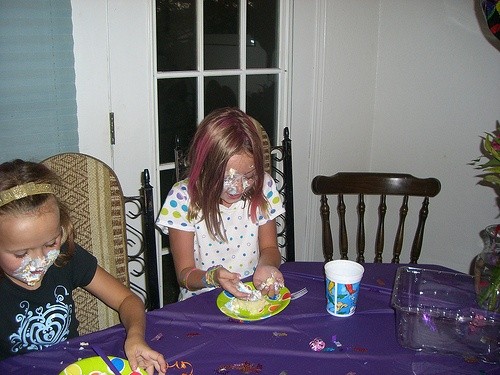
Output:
[311,172,441,264]
[175,126,296,263]
[38,152,161,336]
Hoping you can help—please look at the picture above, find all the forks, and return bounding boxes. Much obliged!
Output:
[291,286,308,300]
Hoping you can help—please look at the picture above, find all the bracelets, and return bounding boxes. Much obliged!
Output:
[204,264,222,289]
[181,266,197,291]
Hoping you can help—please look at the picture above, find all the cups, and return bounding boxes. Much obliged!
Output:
[324,260,365,317]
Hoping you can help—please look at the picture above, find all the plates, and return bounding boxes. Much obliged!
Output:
[217,282,292,321]
[59,356,148,375]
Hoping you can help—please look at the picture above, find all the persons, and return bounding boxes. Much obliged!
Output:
[154,104,288,302]
[0,157,170,375]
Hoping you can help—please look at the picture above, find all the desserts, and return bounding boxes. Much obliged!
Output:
[233,281,267,314]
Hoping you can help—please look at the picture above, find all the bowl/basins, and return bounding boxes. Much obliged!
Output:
[391,265,500,364]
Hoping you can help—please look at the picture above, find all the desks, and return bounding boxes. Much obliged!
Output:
[0,261,500,375]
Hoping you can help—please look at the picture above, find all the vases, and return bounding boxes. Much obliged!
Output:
[473,224,500,316]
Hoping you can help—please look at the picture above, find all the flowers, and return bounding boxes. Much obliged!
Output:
[466,121,500,311]
